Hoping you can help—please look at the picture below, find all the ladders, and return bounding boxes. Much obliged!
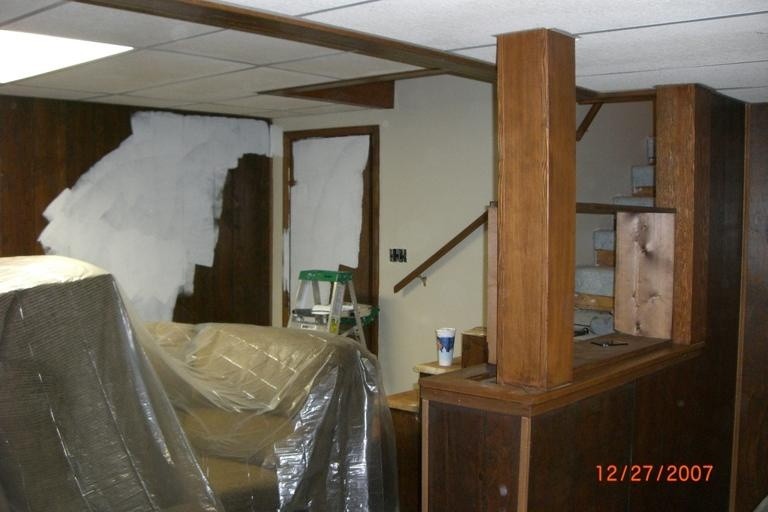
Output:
[287,269,380,347]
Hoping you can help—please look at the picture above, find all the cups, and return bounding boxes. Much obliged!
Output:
[434,326,457,367]
